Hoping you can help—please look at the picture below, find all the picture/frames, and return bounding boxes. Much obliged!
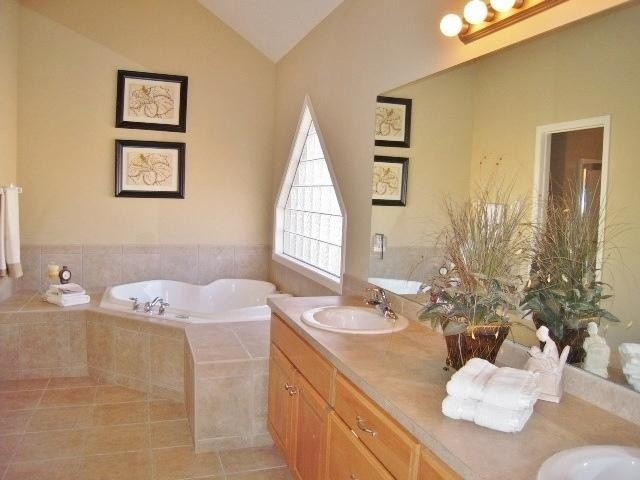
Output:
[113,67,189,133]
[112,138,186,201]
[372,153,410,207]
[375,95,414,149]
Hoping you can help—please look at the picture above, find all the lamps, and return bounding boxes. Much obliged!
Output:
[436,0,569,47]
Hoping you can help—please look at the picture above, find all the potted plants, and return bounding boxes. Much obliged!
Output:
[386,151,536,373]
[516,167,632,365]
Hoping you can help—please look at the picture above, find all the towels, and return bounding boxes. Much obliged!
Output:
[615,341,640,377]
[0,185,25,280]
[47,282,87,297]
[438,394,535,434]
[445,355,541,411]
[621,366,640,394]
[44,290,91,308]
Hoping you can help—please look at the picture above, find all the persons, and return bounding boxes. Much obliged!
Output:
[583,322,607,353]
[525,325,559,372]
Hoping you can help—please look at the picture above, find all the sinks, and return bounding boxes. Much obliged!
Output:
[536,444,640,480]
[300,305,411,335]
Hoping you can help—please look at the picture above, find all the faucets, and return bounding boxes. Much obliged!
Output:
[144,297,165,313]
[373,288,388,309]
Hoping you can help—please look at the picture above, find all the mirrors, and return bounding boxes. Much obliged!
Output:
[361,1,639,399]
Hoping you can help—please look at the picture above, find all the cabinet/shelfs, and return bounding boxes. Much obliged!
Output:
[265,309,340,480]
[326,366,418,480]
[418,443,466,480]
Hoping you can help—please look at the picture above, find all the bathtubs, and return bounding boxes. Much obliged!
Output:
[368,276,432,298]
[98,278,295,324]
